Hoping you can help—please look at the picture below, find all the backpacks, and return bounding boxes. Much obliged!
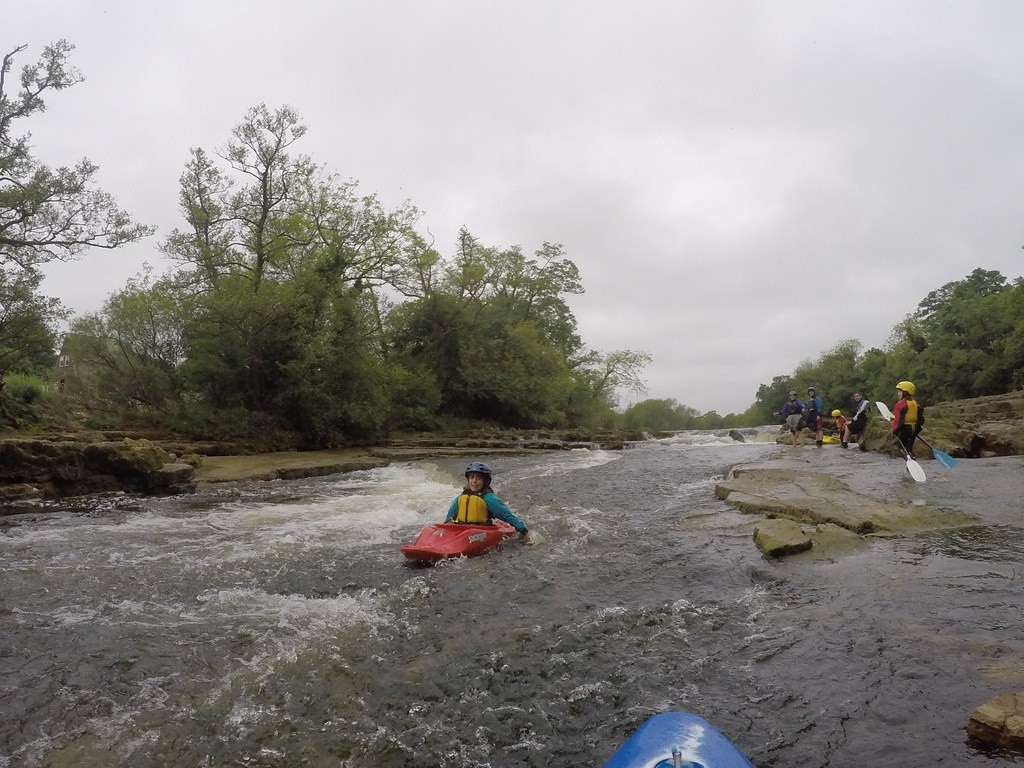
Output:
[788,401,799,415]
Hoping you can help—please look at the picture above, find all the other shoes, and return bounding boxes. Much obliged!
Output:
[841,442,848,448]
[816,440,822,448]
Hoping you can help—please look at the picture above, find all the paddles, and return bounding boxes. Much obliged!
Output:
[888,410,956,470]
[876,401,927,482]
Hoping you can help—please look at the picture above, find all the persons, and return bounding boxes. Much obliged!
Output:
[805,387,824,448]
[891,381,924,471]
[842,390,873,449]
[443,462,531,540]
[831,409,847,441]
[773,391,808,446]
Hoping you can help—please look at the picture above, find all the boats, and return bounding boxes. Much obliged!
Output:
[822,433,843,446]
[400,522,517,559]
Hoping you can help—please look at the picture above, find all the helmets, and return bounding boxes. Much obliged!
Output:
[896,381,916,396]
[808,387,815,396]
[789,391,796,396]
[831,409,841,416]
[465,461,491,475]
